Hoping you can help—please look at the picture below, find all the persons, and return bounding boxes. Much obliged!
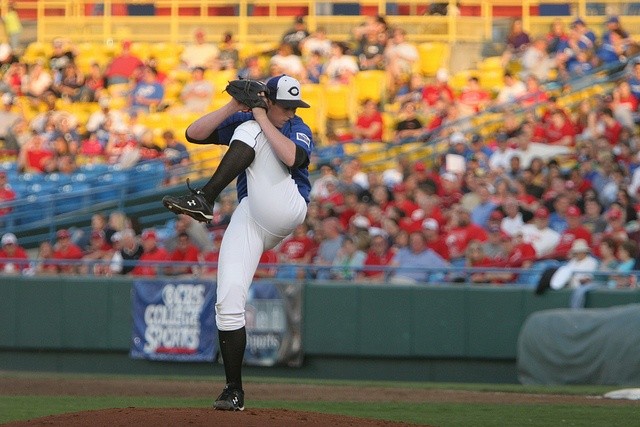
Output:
[1,1,188,185]
[314,64,640,286]
[2,208,313,281]
[623,36,640,60]
[567,15,595,69]
[538,18,584,67]
[499,14,538,64]
[236,10,417,85]
[179,27,220,69]
[211,30,242,72]
[162,73,314,410]
[608,26,627,59]
[179,65,217,115]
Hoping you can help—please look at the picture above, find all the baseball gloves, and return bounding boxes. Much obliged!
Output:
[221,75,270,113]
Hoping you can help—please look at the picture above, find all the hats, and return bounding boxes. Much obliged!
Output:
[264,74,310,110]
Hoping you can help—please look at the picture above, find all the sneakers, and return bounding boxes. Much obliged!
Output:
[213,385,244,411]
[163,178,214,223]
